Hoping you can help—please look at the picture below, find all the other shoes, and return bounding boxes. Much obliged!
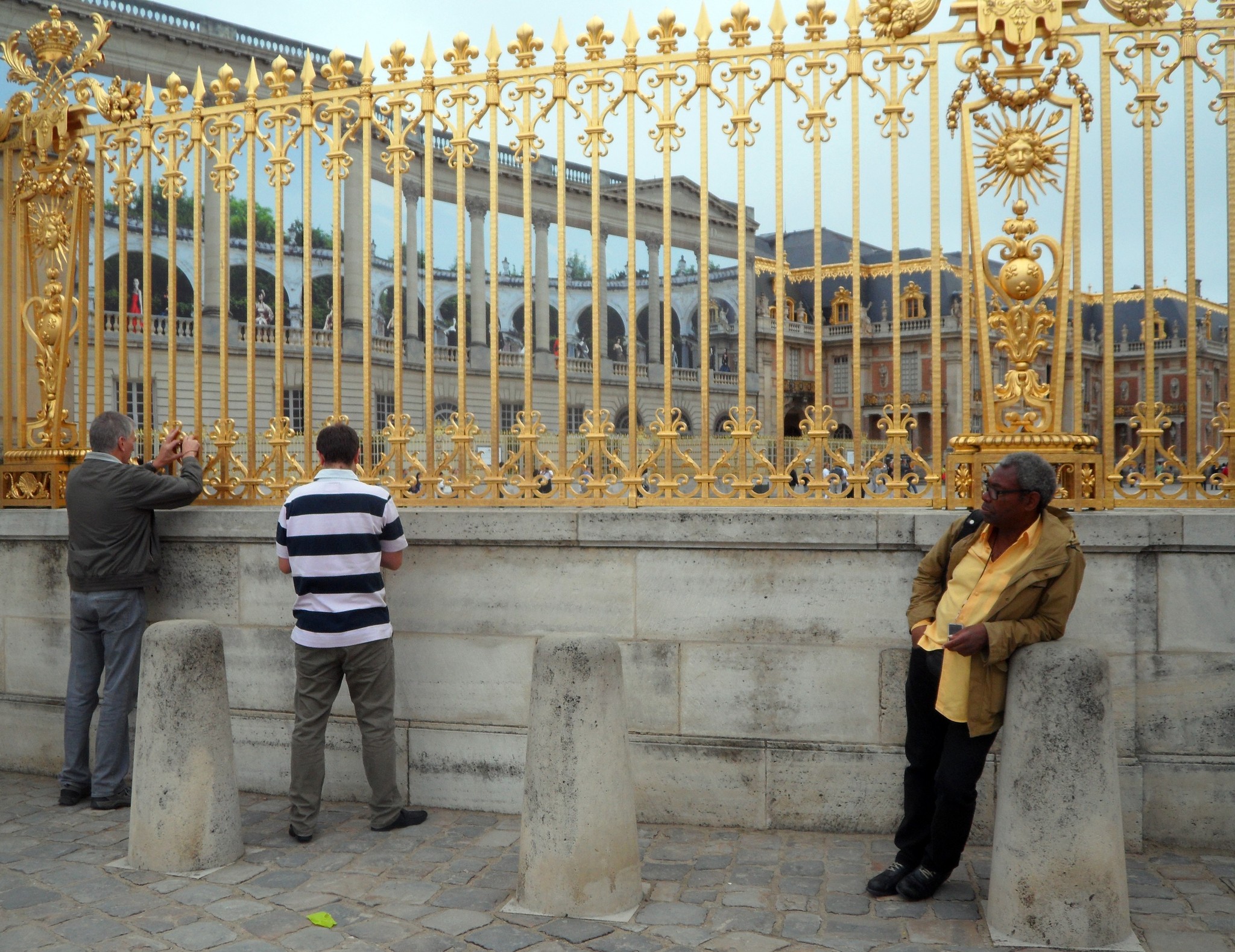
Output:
[289,824,313,841]
[371,810,428,831]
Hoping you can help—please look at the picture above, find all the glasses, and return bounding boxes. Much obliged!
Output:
[981,479,1025,501]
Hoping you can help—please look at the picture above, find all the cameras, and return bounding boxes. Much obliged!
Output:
[947,623,964,641]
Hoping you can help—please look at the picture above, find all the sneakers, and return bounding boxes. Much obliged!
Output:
[866,862,910,895]
[896,866,950,900]
[58,784,83,805]
[90,786,131,809]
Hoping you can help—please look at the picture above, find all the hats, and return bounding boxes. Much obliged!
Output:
[806,459,812,463]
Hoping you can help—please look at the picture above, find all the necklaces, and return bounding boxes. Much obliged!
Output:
[958,531,1023,614]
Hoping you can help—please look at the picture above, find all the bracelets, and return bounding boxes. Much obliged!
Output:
[181,448,198,457]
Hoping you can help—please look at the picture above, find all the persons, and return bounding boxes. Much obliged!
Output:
[576,464,605,508]
[402,468,462,508]
[866,450,1087,903]
[635,464,649,498]
[942,464,945,487]
[521,466,554,509]
[276,424,428,843]
[784,458,920,499]
[1113,461,1229,498]
[496,461,508,509]
[128,276,732,381]
[58,411,203,811]
